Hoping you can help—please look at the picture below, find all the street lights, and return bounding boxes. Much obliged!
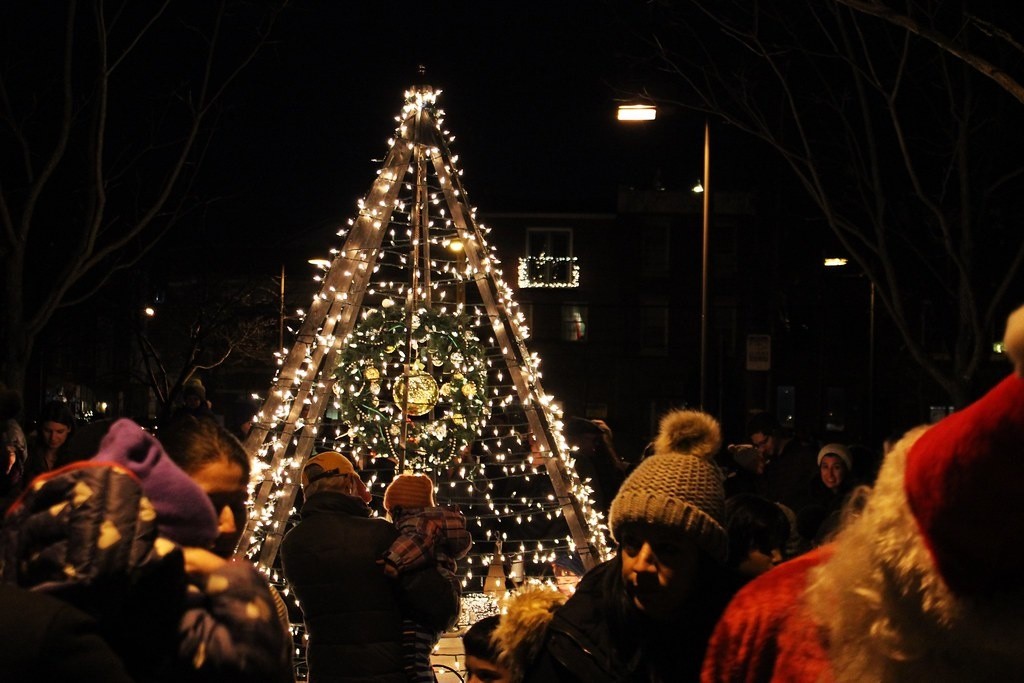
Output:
[612,101,709,414]
[822,253,875,403]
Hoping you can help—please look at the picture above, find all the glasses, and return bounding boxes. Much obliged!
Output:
[754,436,768,449]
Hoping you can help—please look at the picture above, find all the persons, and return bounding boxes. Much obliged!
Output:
[0,373,296,683]
[473,304,1024,683]
[375,470,472,683]
[277,451,460,683]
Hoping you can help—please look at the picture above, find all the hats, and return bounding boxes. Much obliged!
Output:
[568,417,601,436]
[302,451,373,503]
[607,409,729,551]
[728,444,760,466]
[91,417,217,546]
[383,475,433,511]
[817,444,854,472]
[868,305,1024,652]
